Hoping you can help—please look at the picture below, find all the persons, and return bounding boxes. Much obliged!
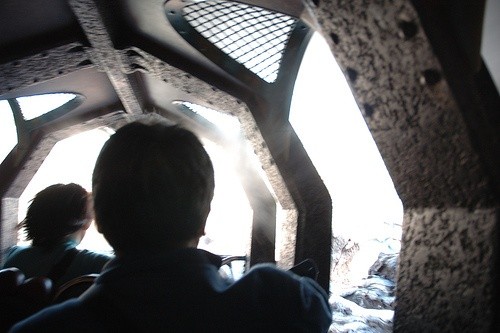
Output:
[10,120,333,333]
[1,182,115,281]
